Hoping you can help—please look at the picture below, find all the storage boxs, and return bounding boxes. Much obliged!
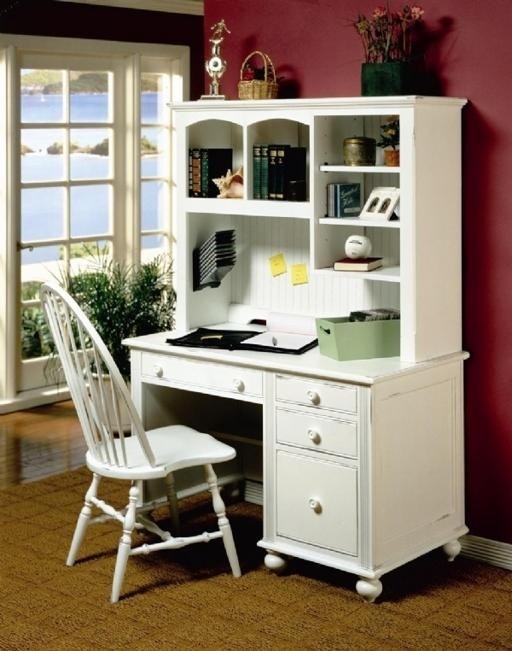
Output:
[315,317,400,361]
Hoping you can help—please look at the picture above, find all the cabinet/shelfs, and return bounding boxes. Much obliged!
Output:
[166,95,467,364]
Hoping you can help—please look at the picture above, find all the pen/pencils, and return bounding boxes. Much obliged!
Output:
[272,337,277,345]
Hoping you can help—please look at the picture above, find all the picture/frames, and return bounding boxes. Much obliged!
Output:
[359,186,400,222]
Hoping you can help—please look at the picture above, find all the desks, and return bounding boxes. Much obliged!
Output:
[121,322,470,602]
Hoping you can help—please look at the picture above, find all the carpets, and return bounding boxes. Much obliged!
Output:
[0,463,512,651]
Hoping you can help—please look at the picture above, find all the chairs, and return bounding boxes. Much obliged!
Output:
[40,282,242,605]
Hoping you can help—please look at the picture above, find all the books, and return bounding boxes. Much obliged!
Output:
[193,149,203,197]
[326,180,364,219]
[199,147,235,198]
[333,255,385,272]
[188,148,194,199]
[252,140,308,202]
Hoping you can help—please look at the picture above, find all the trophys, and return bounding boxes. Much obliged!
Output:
[199,18,232,101]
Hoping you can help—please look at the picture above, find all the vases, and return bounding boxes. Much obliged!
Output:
[361,62,413,94]
[384,150,399,166]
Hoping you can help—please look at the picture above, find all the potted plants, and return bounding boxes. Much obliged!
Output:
[42,241,178,434]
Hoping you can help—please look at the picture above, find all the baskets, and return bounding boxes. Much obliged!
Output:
[237,51,279,100]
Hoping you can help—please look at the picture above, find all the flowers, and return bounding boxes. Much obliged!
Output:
[333,0,425,62]
[375,116,399,150]
